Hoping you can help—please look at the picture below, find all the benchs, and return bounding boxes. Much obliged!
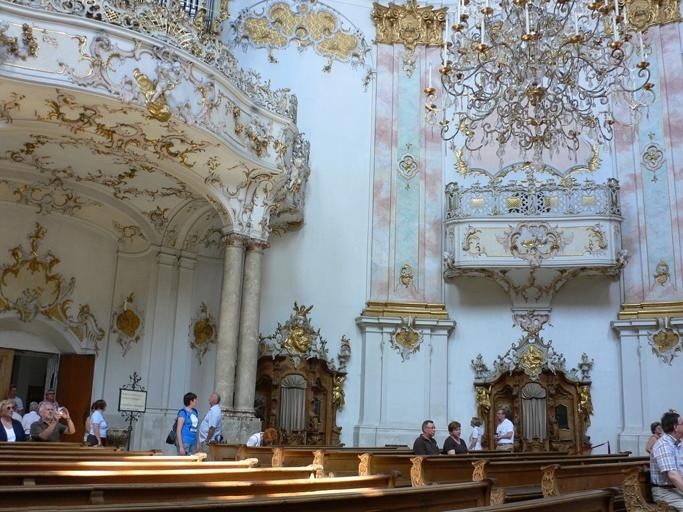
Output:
[1,441,680,511]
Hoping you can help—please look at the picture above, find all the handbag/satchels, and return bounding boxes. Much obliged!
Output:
[166,430,177,444]
[87,434,98,445]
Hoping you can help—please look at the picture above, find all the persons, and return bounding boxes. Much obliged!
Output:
[247,428,278,448]
[198,392,223,461]
[413,420,440,454]
[174,392,200,456]
[650,412,683,512]
[0,385,107,446]
[646,422,662,454]
[469,417,483,450]
[493,409,514,453]
[443,421,469,454]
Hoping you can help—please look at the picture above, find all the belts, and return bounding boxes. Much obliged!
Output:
[653,483,675,488]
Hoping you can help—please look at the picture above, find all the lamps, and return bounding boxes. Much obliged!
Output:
[416,0,658,160]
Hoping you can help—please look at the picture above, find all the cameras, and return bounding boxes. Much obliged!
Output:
[58,407,64,415]
